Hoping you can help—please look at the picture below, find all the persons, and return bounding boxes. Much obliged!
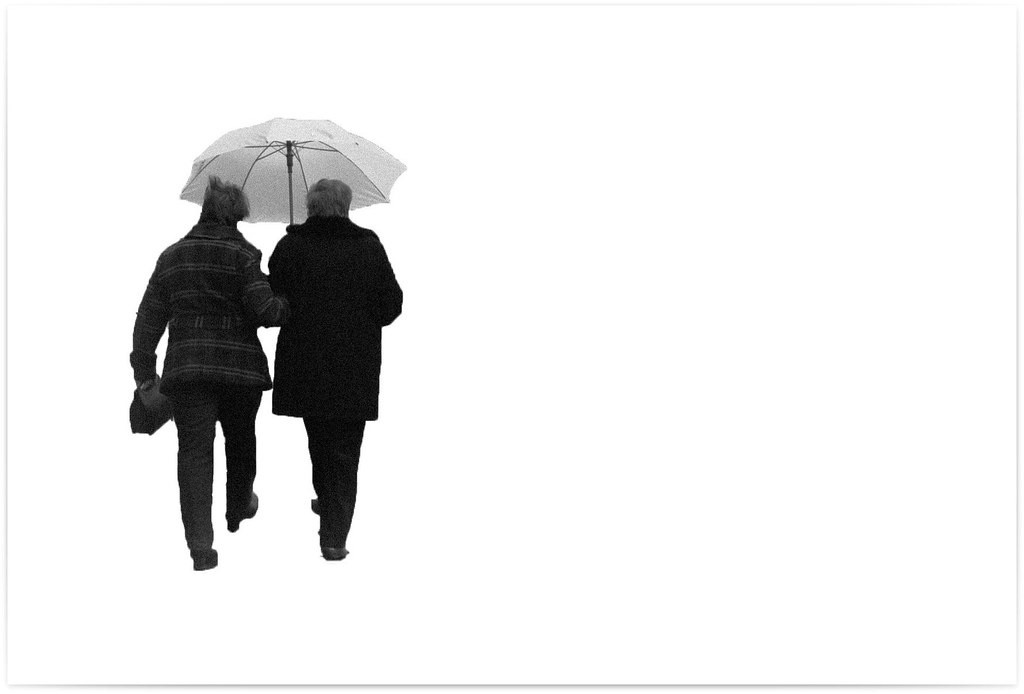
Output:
[129,175,291,571]
[268,178,403,561]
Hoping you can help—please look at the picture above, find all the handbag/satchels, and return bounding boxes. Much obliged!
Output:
[130,379,173,435]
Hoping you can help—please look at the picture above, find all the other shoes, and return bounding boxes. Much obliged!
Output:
[193,548,218,571]
[227,493,258,532]
[311,499,322,515]
[321,546,348,560]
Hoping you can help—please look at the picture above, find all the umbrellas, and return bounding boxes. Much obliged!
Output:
[180,118,408,225]
